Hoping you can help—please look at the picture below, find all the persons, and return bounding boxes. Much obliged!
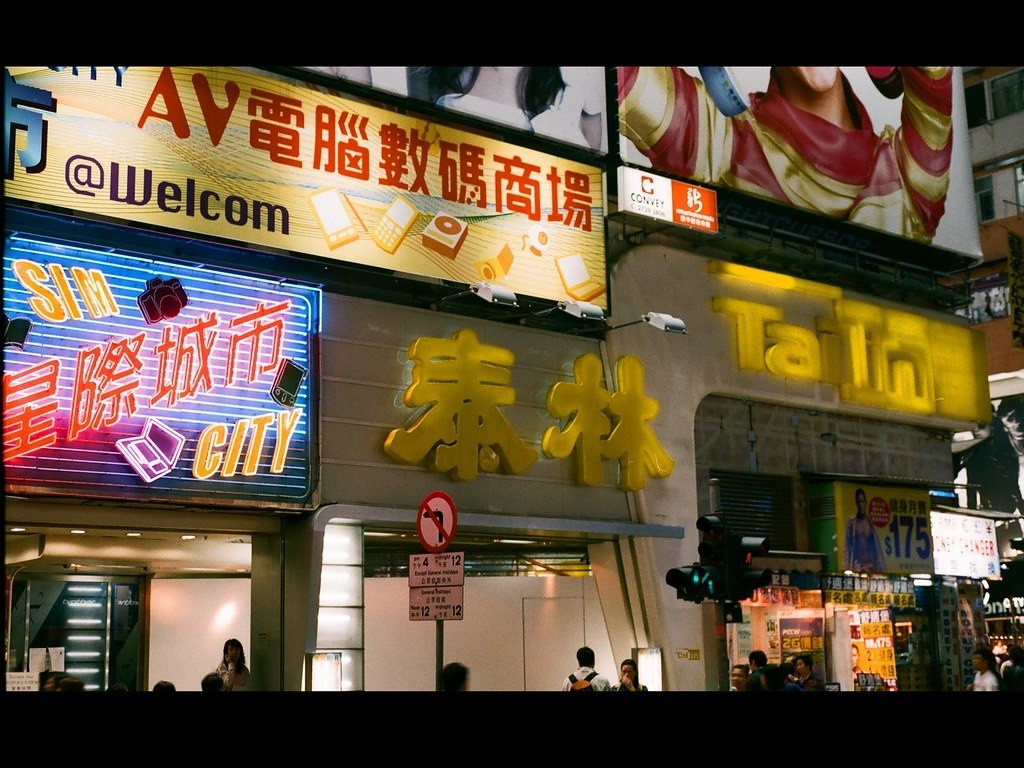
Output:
[964,409,1024,520]
[439,663,471,692]
[846,488,886,574]
[611,659,648,692]
[850,644,863,682]
[727,649,829,694]
[406,66,954,251]
[562,647,612,692]
[42,639,251,695]
[966,639,1024,692]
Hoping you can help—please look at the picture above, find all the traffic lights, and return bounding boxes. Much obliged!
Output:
[696,513,729,601]
[666,563,706,602]
[727,530,774,601]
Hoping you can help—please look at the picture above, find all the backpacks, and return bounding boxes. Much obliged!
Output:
[569,671,598,691]
